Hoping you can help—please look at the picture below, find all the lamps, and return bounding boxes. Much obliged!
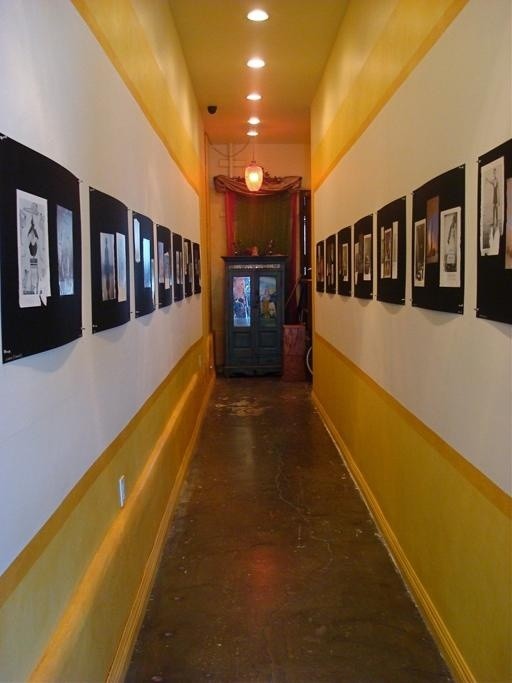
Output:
[245,131,263,193]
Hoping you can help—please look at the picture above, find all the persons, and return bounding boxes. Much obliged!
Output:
[486,168,500,226]
[103,237,111,300]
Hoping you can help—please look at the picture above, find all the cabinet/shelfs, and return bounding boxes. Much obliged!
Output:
[221,254,289,378]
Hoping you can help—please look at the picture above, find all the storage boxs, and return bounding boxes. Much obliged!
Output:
[282,323,306,355]
[282,356,305,381]
